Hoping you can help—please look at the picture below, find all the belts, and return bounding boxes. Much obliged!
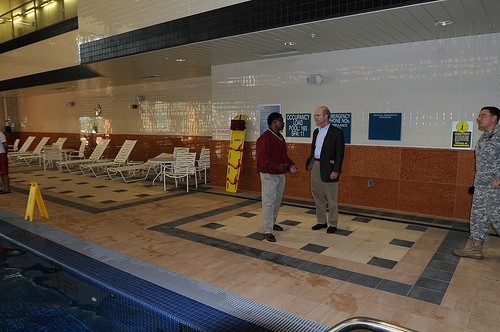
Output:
[313,158,320,162]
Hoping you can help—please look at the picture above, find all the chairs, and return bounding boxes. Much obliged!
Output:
[153,147,212,193]
[40,142,85,173]
[56,139,173,182]
[7,137,67,167]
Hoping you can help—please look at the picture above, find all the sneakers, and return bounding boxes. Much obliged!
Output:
[452,239,485,260]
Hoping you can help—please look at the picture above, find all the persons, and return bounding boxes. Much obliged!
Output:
[453,107,500,260]
[306,106,345,233]
[0,132,11,194]
[255,112,299,242]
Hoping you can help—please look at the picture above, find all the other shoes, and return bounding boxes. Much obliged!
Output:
[263,233,276,242]
[327,226,337,234]
[272,224,283,231]
[311,222,327,230]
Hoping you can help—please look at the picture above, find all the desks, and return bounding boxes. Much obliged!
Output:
[148,158,176,187]
[62,149,73,169]
